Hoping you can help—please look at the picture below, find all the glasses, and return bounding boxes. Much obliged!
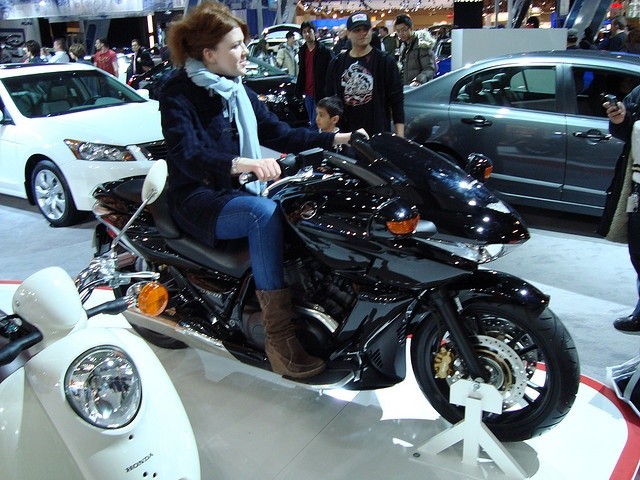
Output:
[393,27,410,33]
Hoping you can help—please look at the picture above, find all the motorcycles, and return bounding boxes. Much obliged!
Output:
[1,161,203,476]
[92,133,580,441]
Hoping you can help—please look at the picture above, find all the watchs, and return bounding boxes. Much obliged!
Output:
[232,157,240,163]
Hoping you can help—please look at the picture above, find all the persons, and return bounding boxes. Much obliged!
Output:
[432,26,448,51]
[579,27,603,50]
[126,39,155,84]
[566,28,582,49]
[68,43,94,66]
[605,16,628,52]
[332,24,352,56]
[23,40,43,63]
[294,21,335,131]
[159,0,370,379]
[276,31,300,81]
[313,96,344,133]
[595,85,640,331]
[379,26,395,60]
[93,37,119,78]
[42,38,70,63]
[394,14,436,87]
[325,12,405,139]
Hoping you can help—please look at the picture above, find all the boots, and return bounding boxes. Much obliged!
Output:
[255,288,326,378]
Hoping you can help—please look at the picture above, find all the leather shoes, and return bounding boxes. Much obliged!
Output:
[613,316,640,335]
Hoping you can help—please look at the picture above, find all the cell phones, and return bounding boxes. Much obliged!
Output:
[603,93,618,110]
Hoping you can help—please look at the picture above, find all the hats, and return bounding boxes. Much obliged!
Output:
[346,13,371,31]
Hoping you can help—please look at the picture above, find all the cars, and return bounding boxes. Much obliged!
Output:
[1,64,166,227]
[390,51,640,218]
[131,57,309,128]
